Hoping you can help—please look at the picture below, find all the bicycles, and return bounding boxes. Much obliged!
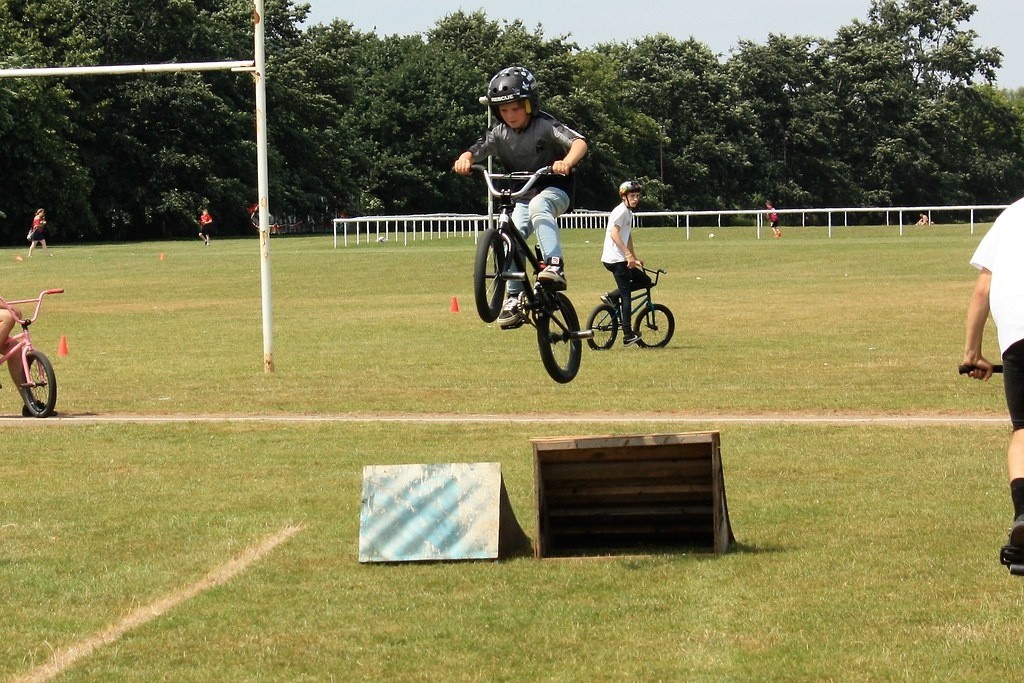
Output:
[586,268,675,351]
[0,289,65,418]
[452,163,595,384]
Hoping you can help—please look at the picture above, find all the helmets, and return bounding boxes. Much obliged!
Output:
[619,181,642,196]
[487,66,539,123]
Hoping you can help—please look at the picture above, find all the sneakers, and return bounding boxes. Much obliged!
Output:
[623,332,642,347]
[600,291,620,317]
[538,257,566,291]
[497,297,522,326]
[22,400,58,417]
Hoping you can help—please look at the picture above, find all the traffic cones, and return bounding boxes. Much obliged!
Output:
[160,252,165,260]
[451,296,460,313]
[16,256,23,261]
[57,335,68,356]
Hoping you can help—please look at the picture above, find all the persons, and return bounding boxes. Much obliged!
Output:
[199,210,213,246]
[28,209,47,256]
[962,197,1024,567]
[767,202,781,238]
[0,304,57,416]
[454,67,587,325]
[920,214,929,225]
[600,182,651,346]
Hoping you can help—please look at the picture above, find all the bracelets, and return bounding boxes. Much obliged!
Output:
[626,254,632,258]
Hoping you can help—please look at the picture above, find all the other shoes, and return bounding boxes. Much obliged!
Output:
[1006,513,1024,570]
[205,240,207,245]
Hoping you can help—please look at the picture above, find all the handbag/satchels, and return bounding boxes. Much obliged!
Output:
[27,229,36,242]
